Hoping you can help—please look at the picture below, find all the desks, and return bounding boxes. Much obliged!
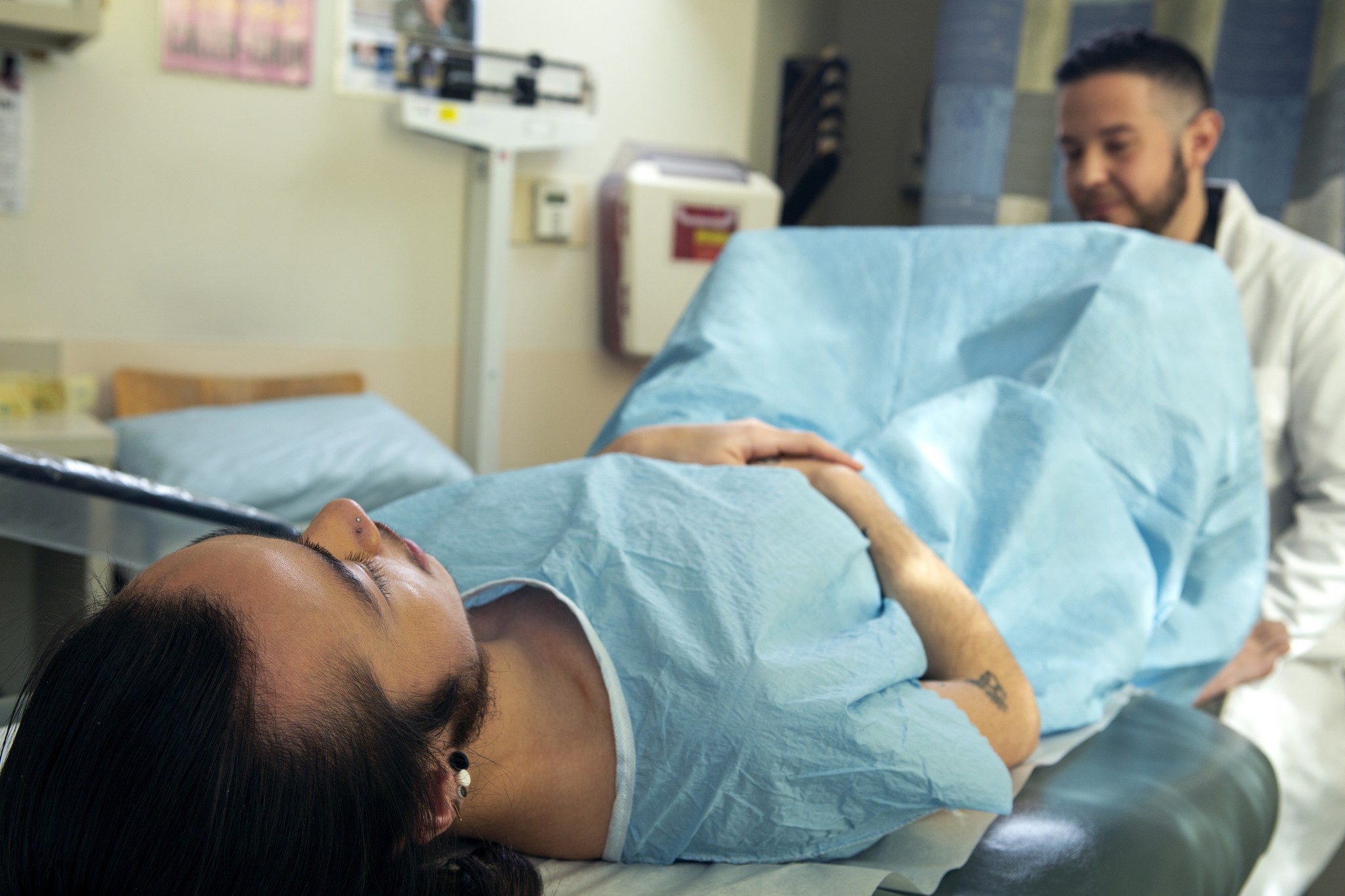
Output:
[1,404,124,617]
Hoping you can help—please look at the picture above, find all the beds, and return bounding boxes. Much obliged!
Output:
[1,445,1345,896]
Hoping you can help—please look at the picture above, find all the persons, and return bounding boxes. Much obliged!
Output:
[0,239,1289,895]
[1052,28,1342,896]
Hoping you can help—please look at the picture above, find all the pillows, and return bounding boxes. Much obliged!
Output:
[113,390,477,522]
